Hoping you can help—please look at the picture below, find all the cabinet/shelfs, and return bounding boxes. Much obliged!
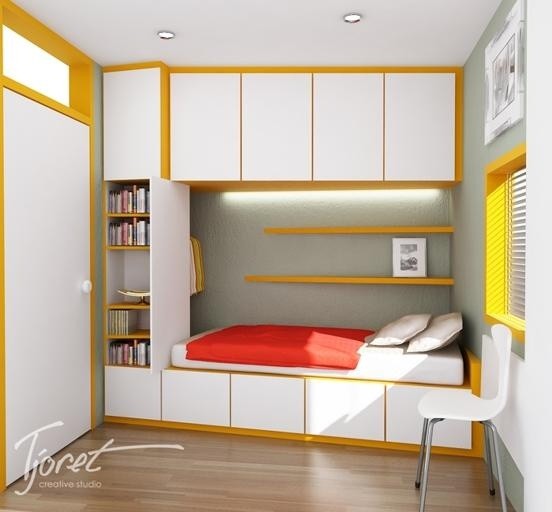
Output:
[171,70,312,185]
[307,378,474,455]
[101,65,168,182]
[162,369,307,439]
[243,227,455,286]
[105,367,162,429]
[312,70,462,184]
[107,180,190,369]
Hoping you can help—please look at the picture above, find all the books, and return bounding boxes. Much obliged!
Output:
[103,185,150,369]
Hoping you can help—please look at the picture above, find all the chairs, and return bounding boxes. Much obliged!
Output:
[413,324,514,512]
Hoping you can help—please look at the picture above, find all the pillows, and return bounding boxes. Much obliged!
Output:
[407,312,464,355]
[366,313,430,347]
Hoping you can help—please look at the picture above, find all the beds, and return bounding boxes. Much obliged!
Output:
[172,312,465,383]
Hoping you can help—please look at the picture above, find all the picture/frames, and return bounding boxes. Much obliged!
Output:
[392,236,428,278]
[484,0,524,147]
